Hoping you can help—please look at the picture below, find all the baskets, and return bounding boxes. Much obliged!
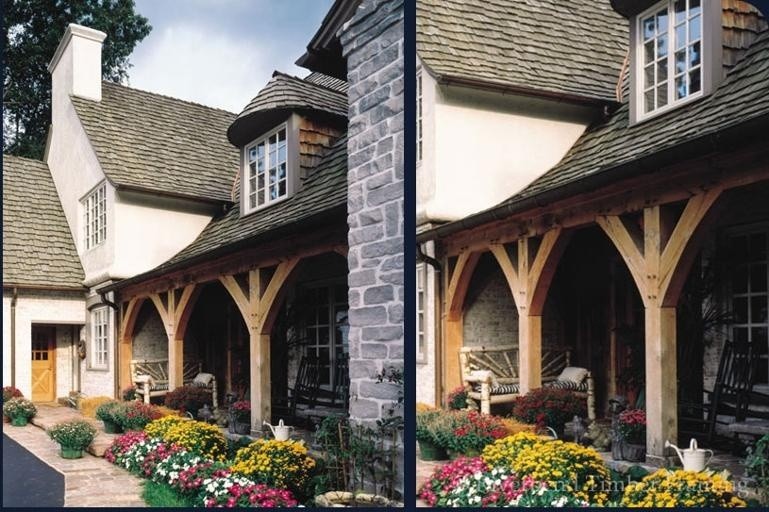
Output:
[612,440,645,462]
[229,422,249,434]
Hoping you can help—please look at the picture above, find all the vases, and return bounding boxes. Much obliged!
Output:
[2,415,29,426]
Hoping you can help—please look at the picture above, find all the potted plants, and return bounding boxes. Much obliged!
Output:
[60,444,84,458]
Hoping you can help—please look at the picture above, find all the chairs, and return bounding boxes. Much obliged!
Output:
[272,355,324,427]
[674,337,762,453]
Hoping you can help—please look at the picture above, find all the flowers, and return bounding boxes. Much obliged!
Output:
[410,381,746,508]
[45,417,100,450]
[96,385,319,510]
[2,383,39,419]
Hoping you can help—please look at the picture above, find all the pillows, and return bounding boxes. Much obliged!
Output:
[556,365,590,386]
[138,374,156,386]
[193,371,214,386]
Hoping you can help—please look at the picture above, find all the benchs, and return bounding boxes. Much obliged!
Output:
[130,357,219,409]
[457,338,599,423]
